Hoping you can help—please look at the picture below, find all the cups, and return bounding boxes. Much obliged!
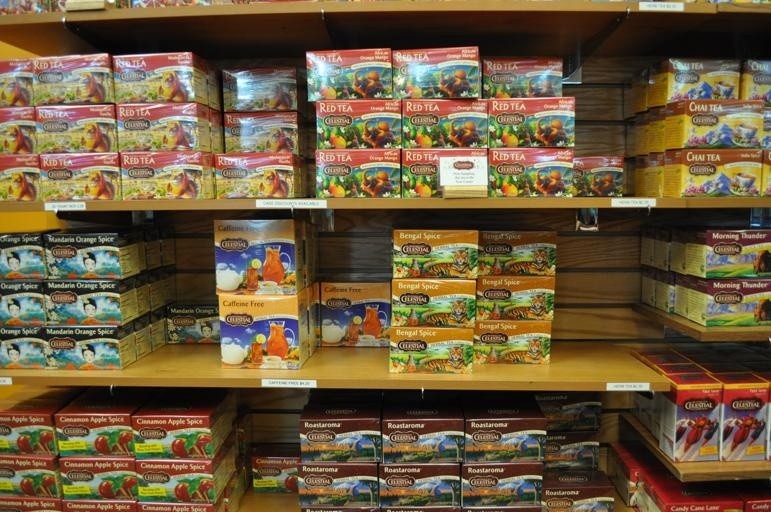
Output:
[251,342,264,364]
[247,268,259,290]
[348,324,359,345]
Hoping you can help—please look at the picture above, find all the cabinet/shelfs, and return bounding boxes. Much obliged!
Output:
[1,0,771,511]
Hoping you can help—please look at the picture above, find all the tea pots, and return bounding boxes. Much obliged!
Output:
[266,321,295,360]
[222,343,249,365]
[262,244,284,286]
[363,305,387,340]
[215,268,243,291]
[322,324,347,343]
